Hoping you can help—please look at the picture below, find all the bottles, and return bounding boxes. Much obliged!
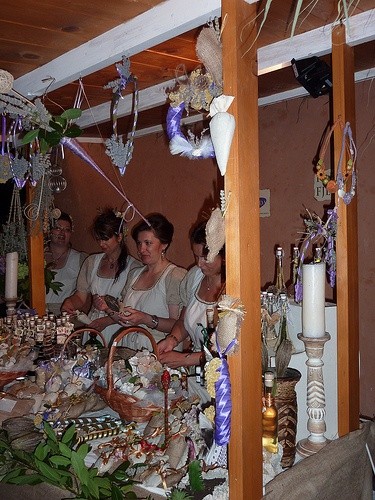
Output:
[261,372,278,454]
[273,248,286,289]
[3,311,102,382]
[261,292,295,354]
[287,247,300,296]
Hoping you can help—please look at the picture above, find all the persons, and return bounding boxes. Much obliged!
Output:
[44,212,88,303]
[60,207,143,346]
[91,214,188,353]
[154,220,226,369]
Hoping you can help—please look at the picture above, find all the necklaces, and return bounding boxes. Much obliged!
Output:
[108,256,118,269]
[206,276,217,291]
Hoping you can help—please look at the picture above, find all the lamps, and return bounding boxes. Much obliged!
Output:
[290,55,333,98]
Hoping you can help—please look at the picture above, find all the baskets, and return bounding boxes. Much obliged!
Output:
[97,326,163,422]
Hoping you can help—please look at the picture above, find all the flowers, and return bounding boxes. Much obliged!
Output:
[0,256,63,302]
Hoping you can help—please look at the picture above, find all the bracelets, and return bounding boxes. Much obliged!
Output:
[147,315,159,329]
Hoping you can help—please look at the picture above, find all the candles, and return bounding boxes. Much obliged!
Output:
[5,252,18,299]
[302,261,325,339]
